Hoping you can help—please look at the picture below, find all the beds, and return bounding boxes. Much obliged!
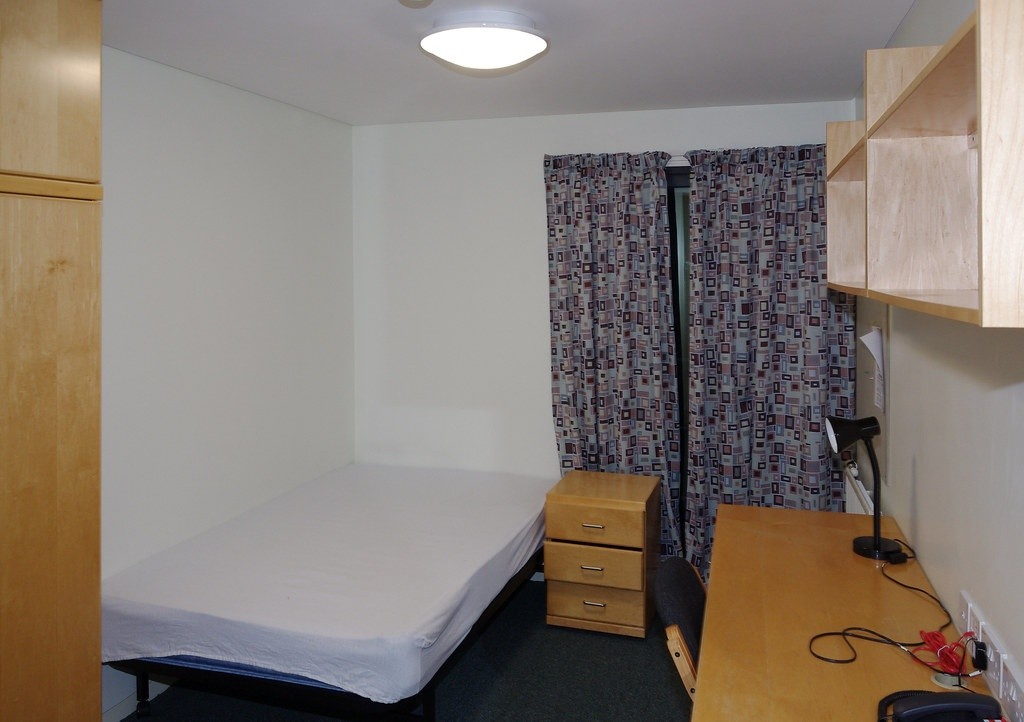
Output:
[100,460,558,722]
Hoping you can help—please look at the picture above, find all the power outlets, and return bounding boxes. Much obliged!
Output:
[953,591,1024,722]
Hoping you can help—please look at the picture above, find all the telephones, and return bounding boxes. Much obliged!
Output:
[875,690,1004,722]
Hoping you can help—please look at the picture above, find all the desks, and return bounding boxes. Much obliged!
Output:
[688,502,1007,722]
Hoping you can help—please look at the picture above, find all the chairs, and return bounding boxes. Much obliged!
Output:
[655,553,707,703]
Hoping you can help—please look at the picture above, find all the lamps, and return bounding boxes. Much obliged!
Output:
[417,9,548,73]
[823,413,906,562]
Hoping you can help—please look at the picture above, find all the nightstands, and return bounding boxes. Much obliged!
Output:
[543,470,662,639]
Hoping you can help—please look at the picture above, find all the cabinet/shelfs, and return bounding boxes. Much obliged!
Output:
[0,2,100,722]
[823,0,1024,329]
